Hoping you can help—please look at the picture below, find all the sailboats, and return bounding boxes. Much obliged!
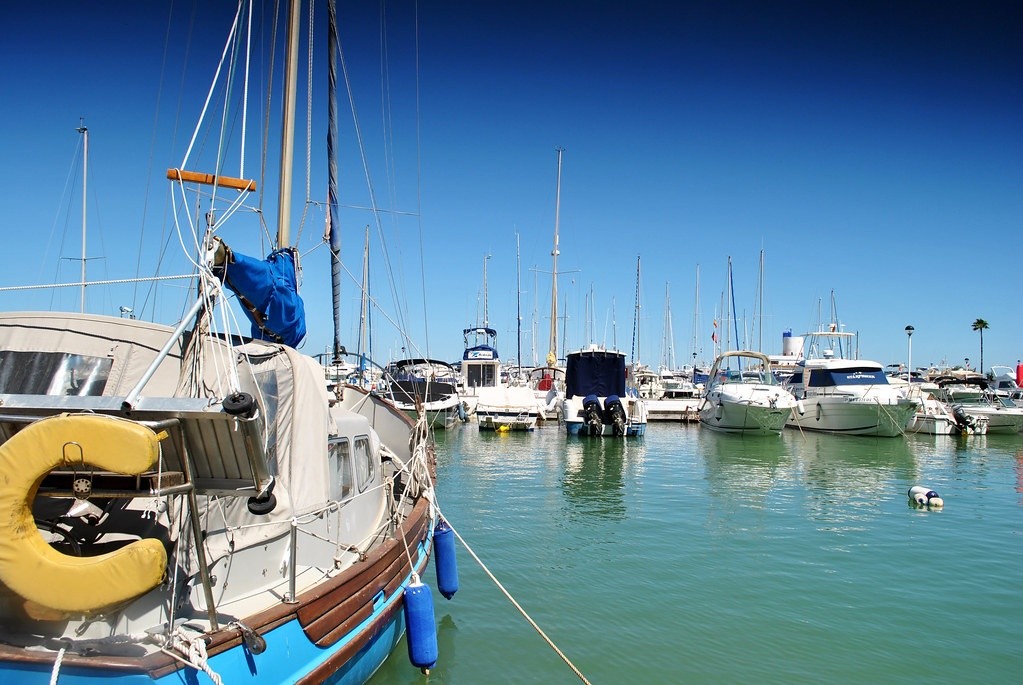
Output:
[16,1,1023,439]
[0,1,460,685]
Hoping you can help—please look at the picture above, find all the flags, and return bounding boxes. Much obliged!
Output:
[713,319,718,329]
[711,332,718,343]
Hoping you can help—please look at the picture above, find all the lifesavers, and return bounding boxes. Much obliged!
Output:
[0,411,169,614]
